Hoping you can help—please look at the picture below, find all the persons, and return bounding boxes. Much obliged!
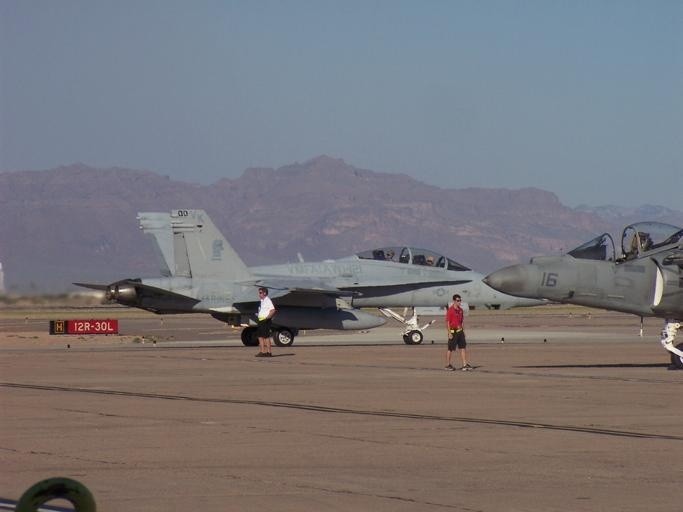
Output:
[427,256,435,265]
[255,287,276,357]
[445,294,474,371]
[387,250,395,259]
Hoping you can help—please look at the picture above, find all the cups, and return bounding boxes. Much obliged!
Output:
[455,299,461,302]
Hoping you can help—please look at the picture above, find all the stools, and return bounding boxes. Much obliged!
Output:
[450,327,463,333]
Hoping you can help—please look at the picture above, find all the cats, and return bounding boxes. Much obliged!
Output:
[69,206,557,345]
[481,219,682,370]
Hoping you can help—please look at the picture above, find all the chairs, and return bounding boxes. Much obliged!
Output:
[444,365,455,371]
[461,363,473,371]
[254,351,271,357]
